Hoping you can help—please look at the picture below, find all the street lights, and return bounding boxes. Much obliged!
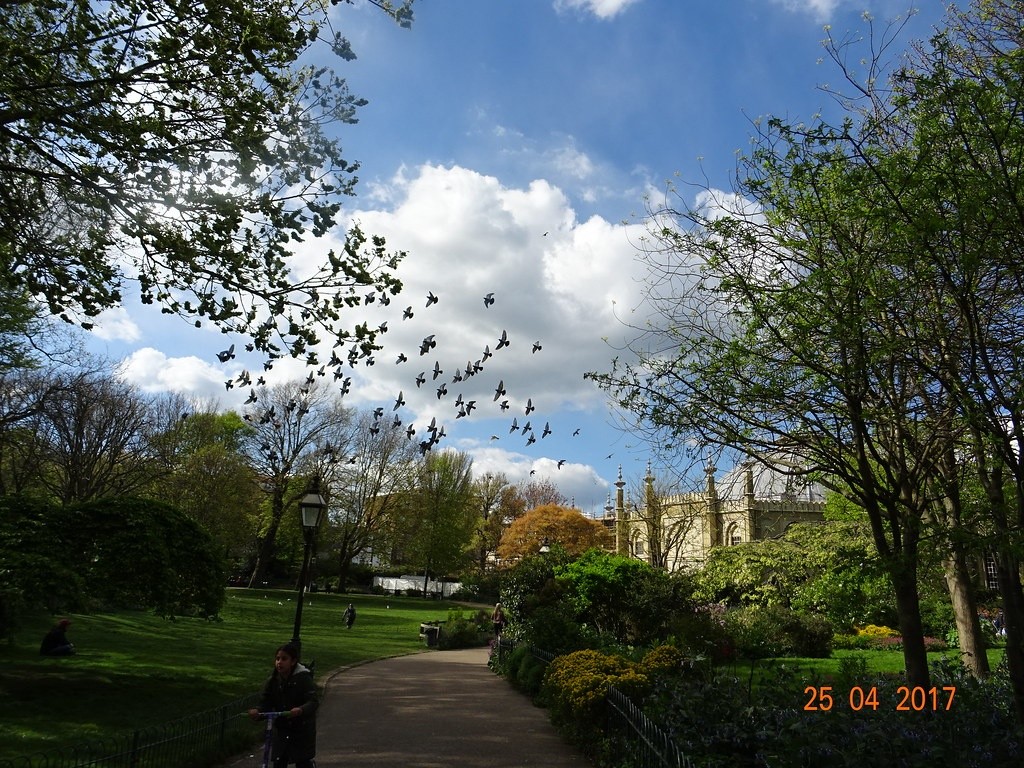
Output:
[287,474,329,662]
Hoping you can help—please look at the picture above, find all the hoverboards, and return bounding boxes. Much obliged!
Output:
[236,710,318,768]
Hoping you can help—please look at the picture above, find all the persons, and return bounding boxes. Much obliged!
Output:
[249,644,320,768]
[342,604,357,629]
[40,619,79,656]
[491,603,507,637]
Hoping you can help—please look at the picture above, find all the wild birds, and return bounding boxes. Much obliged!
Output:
[605,454,614,460]
[216,290,581,477]
[543,232,548,236]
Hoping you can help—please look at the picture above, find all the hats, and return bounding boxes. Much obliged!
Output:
[59,619,70,625]
